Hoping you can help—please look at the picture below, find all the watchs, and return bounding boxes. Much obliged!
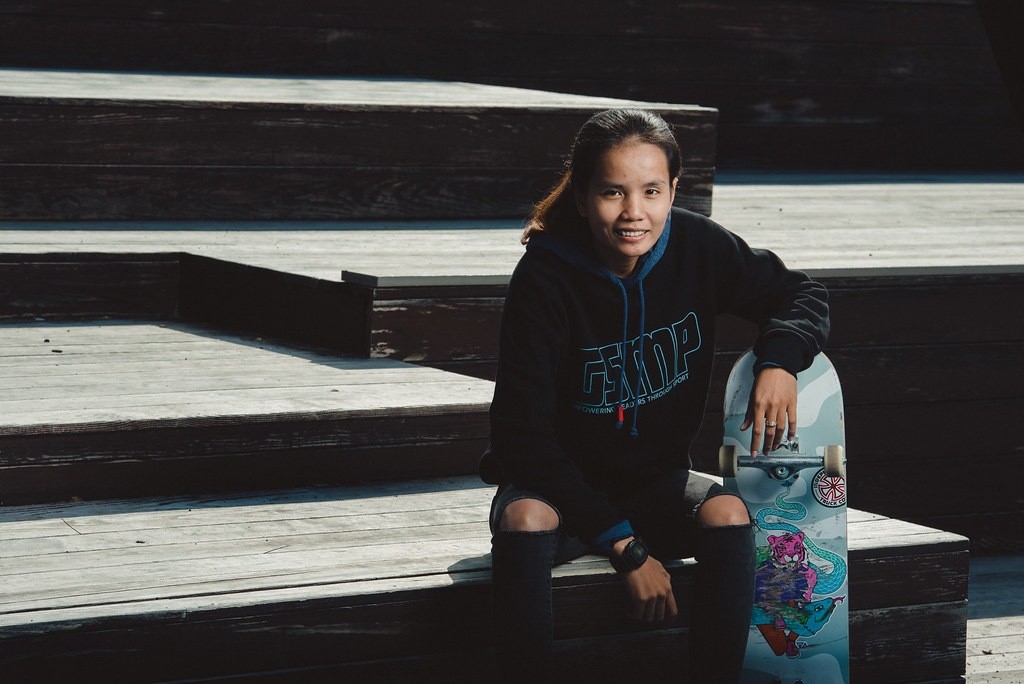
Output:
[612,536,649,576]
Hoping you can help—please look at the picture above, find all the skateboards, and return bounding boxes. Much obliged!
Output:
[718,343,850,683]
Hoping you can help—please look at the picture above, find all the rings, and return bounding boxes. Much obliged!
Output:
[765,421,777,426]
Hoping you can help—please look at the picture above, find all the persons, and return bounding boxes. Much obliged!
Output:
[478,109,831,684]
